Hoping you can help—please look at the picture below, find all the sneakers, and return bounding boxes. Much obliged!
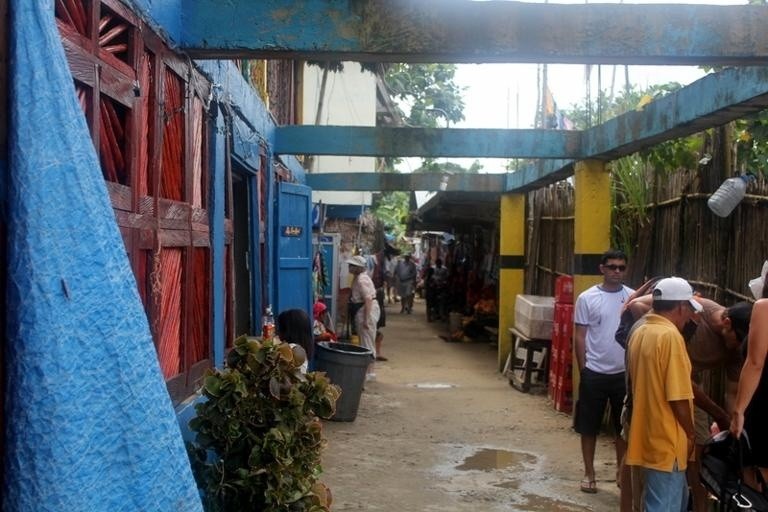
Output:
[388,297,416,315]
[376,356,388,361]
[439,334,460,343]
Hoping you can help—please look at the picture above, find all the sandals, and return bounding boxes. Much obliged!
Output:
[579,479,597,493]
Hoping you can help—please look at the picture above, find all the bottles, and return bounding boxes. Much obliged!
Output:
[262,308,276,343]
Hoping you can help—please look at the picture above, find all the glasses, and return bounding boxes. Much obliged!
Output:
[602,263,626,272]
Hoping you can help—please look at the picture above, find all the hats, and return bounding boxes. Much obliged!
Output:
[345,256,369,268]
[652,277,703,313]
[403,250,412,257]
[728,302,752,343]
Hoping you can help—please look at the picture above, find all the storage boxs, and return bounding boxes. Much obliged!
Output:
[514,293,555,339]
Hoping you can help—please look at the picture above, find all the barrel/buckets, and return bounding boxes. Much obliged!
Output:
[316,340,373,423]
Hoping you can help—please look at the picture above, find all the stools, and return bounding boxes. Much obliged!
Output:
[508,328,548,394]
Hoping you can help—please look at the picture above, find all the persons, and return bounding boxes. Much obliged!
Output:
[274,309,314,385]
[312,302,336,344]
[385,251,497,344]
[572,249,638,493]
[614,259,767,511]
[343,254,381,381]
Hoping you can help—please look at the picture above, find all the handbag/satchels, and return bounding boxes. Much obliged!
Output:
[694,427,767,512]
